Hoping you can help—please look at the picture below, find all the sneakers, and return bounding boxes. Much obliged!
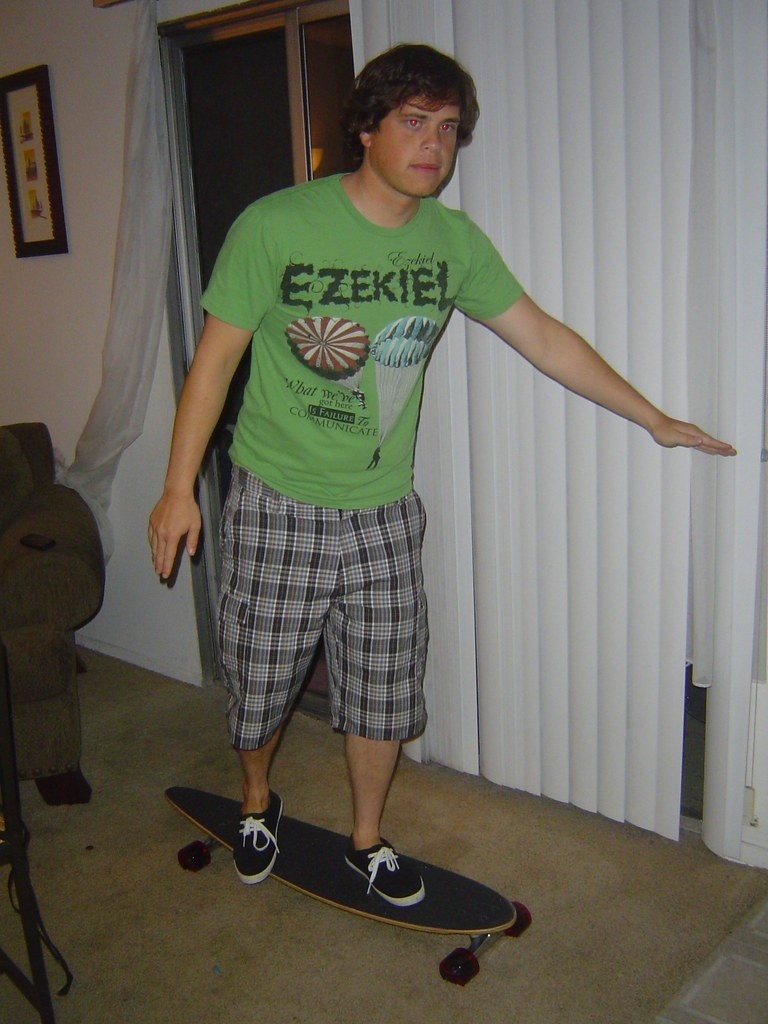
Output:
[344,833,425,907]
[233,789,283,884]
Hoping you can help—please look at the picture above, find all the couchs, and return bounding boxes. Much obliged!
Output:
[0,421,106,805]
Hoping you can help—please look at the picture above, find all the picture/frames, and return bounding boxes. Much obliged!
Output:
[0,64,68,259]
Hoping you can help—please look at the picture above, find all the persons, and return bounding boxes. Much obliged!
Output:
[149,42,736,908]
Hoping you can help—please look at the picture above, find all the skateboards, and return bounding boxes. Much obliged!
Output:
[158,776,536,988]
